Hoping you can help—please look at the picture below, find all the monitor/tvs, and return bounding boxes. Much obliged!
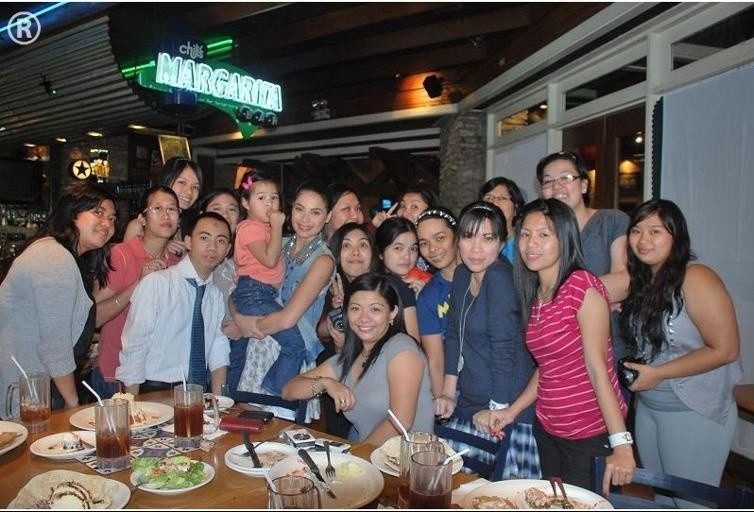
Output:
[0,157,41,206]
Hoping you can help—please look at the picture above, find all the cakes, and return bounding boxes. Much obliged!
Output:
[48,481,95,509]
[49,431,85,451]
[113,392,147,425]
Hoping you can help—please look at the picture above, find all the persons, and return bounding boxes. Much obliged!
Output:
[0,147,744,508]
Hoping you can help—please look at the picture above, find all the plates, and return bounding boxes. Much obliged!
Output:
[462,478,616,509]
[68,400,177,429]
[27,429,98,462]
[8,469,132,509]
[129,460,216,495]
[369,438,465,478]
[224,441,294,477]
[266,451,386,509]
[203,396,234,416]
[0,421,28,455]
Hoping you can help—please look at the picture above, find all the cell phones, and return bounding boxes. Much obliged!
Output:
[314,437,351,453]
[380,195,391,214]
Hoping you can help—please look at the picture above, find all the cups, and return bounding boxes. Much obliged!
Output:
[94,399,131,468]
[264,476,322,509]
[6,372,53,427]
[408,451,453,509]
[173,383,220,450]
[397,430,445,508]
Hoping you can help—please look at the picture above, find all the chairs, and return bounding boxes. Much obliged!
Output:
[590,454,754,509]
[433,405,512,482]
[220,383,308,428]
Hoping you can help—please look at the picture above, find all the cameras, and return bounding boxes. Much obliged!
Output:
[328,306,345,333]
[284,429,315,448]
[618,356,646,386]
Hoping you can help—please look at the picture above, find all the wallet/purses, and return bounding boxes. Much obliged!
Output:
[219,415,265,433]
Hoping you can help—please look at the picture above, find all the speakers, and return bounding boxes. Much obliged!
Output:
[423,74,443,98]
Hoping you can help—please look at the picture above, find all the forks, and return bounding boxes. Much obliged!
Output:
[324,440,335,480]
[556,476,574,509]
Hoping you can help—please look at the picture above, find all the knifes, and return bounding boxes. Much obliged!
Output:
[296,447,337,500]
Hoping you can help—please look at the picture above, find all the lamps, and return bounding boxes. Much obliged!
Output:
[423,74,447,98]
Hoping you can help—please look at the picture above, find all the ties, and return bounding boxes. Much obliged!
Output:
[185,278,208,393]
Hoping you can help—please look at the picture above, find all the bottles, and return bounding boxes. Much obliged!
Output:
[27,211,47,228]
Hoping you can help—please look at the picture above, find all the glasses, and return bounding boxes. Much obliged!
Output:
[143,205,182,215]
[540,175,581,189]
